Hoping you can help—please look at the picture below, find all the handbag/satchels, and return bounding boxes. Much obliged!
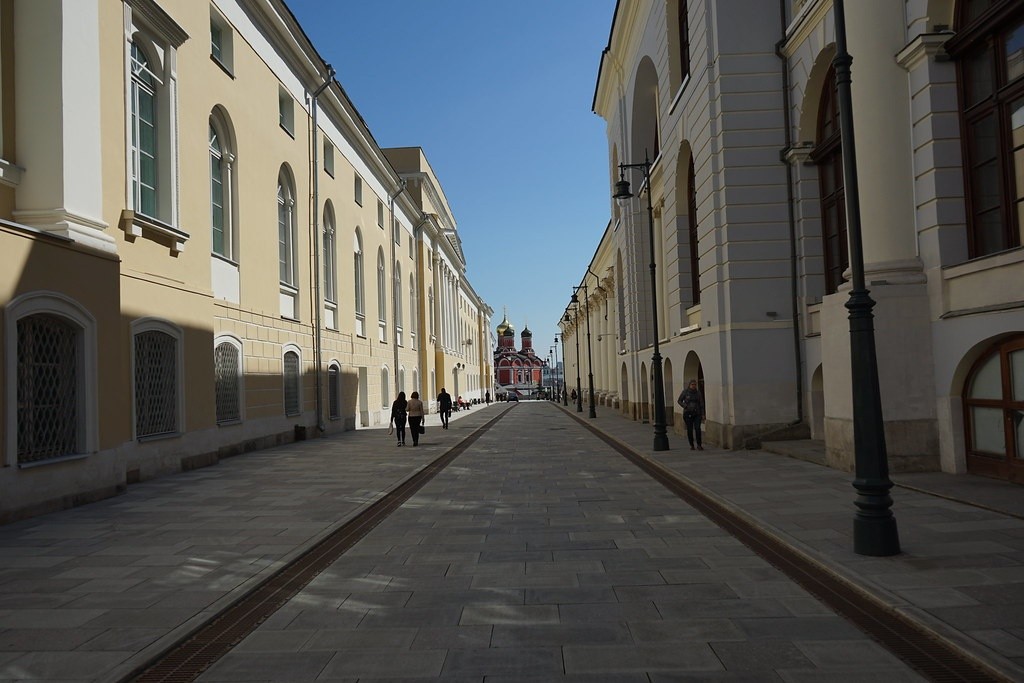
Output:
[419,425,425,434]
[448,411,451,417]
[388,422,393,435]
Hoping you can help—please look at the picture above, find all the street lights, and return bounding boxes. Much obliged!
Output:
[569,280,598,419]
[563,307,583,412]
[611,146,670,450]
[553,331,568,407]
[549,346,561,405]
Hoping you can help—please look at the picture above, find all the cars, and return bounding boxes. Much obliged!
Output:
[507,393,519,403]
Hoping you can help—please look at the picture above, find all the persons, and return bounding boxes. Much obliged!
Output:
[405,392,425,446]
[484,390,490,406]
[571,388,577,404]
[457,396,470,410]
[495,392,504,402]
[437,388,452,429]
[562,390,568,400]
[391,391,407,447]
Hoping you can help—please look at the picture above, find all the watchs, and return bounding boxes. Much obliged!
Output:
[677,380,706,451]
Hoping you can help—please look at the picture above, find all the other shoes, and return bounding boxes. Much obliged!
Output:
[697,446,704,450]
[443,423,445,428]
[396,441,401,446]
[690,446,695,450]
[413,442,418,446]
[446,427,448,429]
[402,441,405,445]
